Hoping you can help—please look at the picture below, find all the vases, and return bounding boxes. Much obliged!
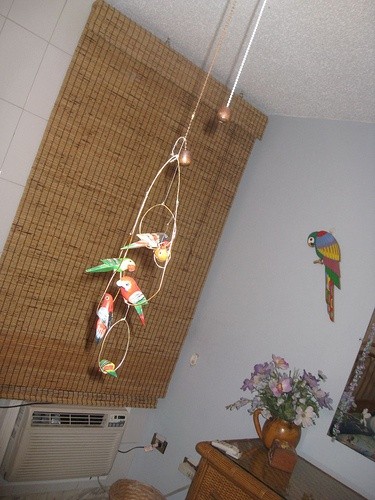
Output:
[253,408,302,451]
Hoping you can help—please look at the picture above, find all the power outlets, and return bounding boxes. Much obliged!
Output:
[150,433,168,455]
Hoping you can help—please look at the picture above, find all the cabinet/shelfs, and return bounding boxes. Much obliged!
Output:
[180,438,372,499]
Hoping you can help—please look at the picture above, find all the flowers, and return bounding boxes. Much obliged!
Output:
[225,354,335,430]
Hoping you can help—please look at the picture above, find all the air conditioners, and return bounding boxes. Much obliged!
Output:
[1,403,127,482]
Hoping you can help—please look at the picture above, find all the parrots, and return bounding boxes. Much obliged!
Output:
[99,359,118,377]
[120,233,168,250]
[116,276,148,327]
[84,258,136,273]
[94,292,113,344]
